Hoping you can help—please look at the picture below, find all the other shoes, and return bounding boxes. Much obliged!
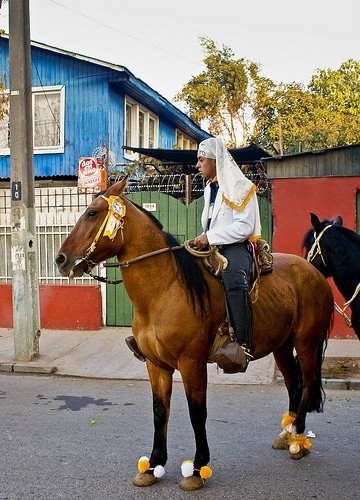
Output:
[212,342,252,374]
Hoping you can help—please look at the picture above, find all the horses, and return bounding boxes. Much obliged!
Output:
[302,212,360,342]
[55,176,334,490]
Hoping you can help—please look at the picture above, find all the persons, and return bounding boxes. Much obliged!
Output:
[125,138,262,373]
